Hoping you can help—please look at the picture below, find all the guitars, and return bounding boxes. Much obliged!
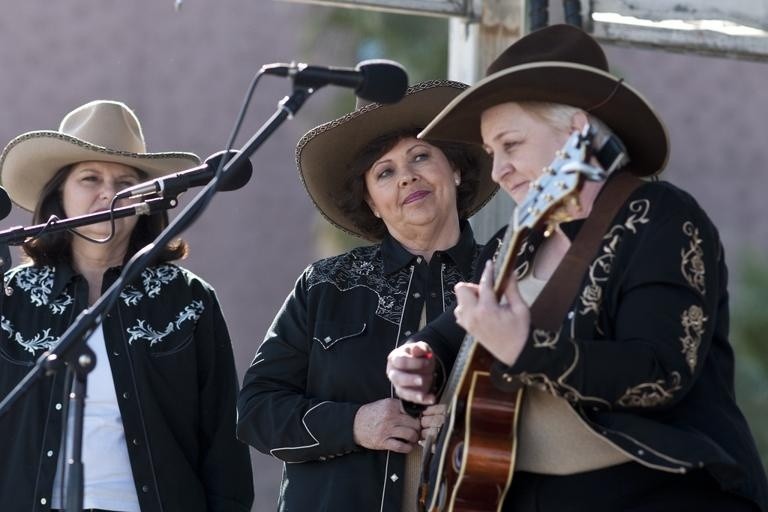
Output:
[416,122,627,512]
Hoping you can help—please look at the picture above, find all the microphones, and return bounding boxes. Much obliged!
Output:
[115,149,254,200]
[263,58,410,105]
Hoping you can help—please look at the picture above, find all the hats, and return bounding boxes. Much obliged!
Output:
[0,99,202,214]
[295,79,500,244]
[416,24,670,177]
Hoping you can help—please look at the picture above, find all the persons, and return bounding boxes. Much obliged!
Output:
[0,99,255,512]
[235,79,502,512]
[386,24,767,512]
